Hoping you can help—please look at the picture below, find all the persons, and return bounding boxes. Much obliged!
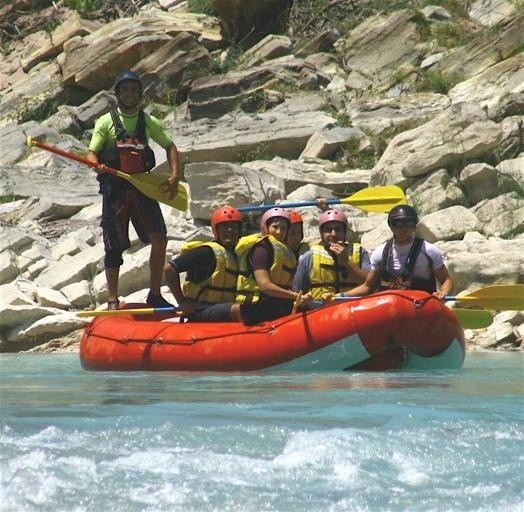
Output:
[291,210,370,315]
[285,197,332,264]
[86,70,180,311]
[321,204,453,303]
[233,207,305,325]
[163,205,241,324]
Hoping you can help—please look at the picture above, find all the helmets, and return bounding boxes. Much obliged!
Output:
[211,205,242,242]
[287,210,303,241]
[319,209,348,242]
[260,207,290,241]
[388,205,418,228]
[114,70,142,95]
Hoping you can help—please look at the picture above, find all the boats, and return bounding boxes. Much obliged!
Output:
[79,279,471,378]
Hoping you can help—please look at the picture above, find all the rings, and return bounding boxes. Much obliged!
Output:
[435,294,438,296]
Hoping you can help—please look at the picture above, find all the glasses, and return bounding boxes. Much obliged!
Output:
[393,221,417,227]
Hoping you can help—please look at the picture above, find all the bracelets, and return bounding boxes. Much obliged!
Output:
[340,291,345,297]
[179,297,185,306]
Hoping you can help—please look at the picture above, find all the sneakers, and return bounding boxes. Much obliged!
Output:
[147,294,174,307]
[107,297,120,311]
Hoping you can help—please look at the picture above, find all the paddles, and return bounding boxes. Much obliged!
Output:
[225,184,407,213]
[329,284,524,310]
[28,135,188,212]
[293,289,493,328]
[75,304,209,316]
